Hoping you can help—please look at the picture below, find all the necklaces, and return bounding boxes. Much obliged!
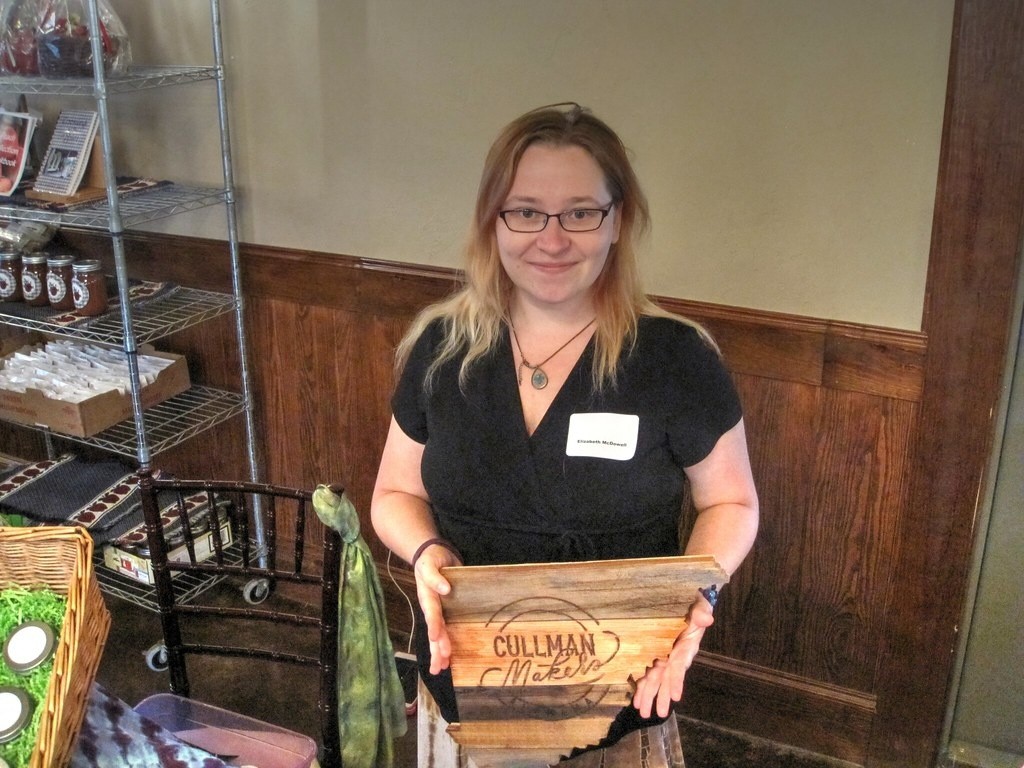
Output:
[507,286,598,389]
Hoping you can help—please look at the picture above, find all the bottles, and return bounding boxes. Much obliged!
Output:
[170,531,184,551]
[206,507,227,530]
[123,544,136,555]
[22,252,51,306]
[189,517,210,540]
[138,543,151,559]
[71,259,107,316]
[46,255,78,310]
[0,250,22,302]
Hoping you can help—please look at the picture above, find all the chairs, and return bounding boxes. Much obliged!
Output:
[133,465,347,768]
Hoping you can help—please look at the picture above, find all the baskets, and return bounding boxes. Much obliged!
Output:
[0,527,110,768]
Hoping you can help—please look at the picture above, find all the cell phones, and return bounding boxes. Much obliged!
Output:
[393,651,422,708]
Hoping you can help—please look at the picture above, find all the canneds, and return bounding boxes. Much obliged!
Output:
[0,252,110,316]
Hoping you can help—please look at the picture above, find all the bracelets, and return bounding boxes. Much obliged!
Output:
[702,586,721,606]
[411,539,463,565]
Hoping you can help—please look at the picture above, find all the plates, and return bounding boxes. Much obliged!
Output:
[133,693,318,768]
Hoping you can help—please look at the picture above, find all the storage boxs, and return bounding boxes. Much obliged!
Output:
[102,516,234,588]
[0,337,191,438]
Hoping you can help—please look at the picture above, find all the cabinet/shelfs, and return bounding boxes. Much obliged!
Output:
[0,0,270,668]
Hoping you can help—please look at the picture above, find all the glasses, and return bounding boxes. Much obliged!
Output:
[498,200,611,231]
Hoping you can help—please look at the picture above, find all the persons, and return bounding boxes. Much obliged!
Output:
[370,103,760,768]
